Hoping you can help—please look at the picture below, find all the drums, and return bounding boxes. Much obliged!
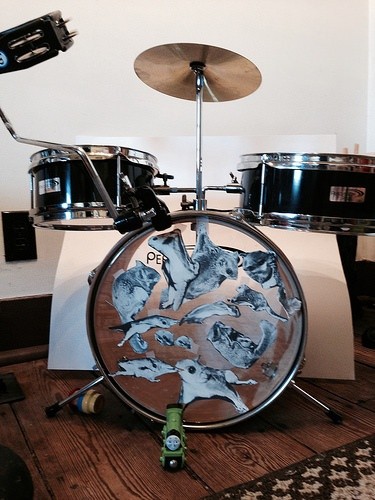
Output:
[28,144,158,232]
[236,151,375,237]
[87,208,309,431]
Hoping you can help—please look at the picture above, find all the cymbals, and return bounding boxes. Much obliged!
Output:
[0,10,79,74]
[132,41,263,103]
